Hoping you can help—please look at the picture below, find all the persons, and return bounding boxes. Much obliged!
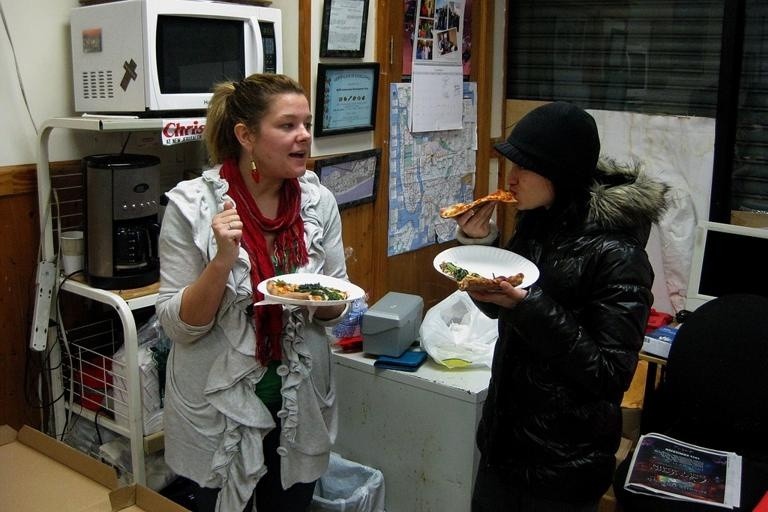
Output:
[455,101,668,512]
[156,73,352,512]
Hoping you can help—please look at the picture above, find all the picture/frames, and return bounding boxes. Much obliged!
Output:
[313,1,383,211]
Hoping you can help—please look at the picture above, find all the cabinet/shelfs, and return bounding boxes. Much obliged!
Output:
[33,114,209,493]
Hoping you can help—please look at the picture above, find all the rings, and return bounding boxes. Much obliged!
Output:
[228,223,234,230]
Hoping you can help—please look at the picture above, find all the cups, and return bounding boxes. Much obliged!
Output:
[60,231,86,278]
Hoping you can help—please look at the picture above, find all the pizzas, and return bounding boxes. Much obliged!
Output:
[440,188,518,219]
[440,261,524,292]
[267,280,349,301]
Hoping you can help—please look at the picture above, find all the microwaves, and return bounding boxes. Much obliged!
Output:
[70,1,283,117]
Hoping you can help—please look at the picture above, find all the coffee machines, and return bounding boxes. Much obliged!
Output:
[83,154,162,290]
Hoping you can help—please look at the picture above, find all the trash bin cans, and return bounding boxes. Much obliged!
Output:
[311,450,392,507]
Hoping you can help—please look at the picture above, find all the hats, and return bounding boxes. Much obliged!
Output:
[491,100,601,186]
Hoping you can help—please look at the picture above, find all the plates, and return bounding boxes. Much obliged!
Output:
[256,274,366,305]
[433,244,542,291]
